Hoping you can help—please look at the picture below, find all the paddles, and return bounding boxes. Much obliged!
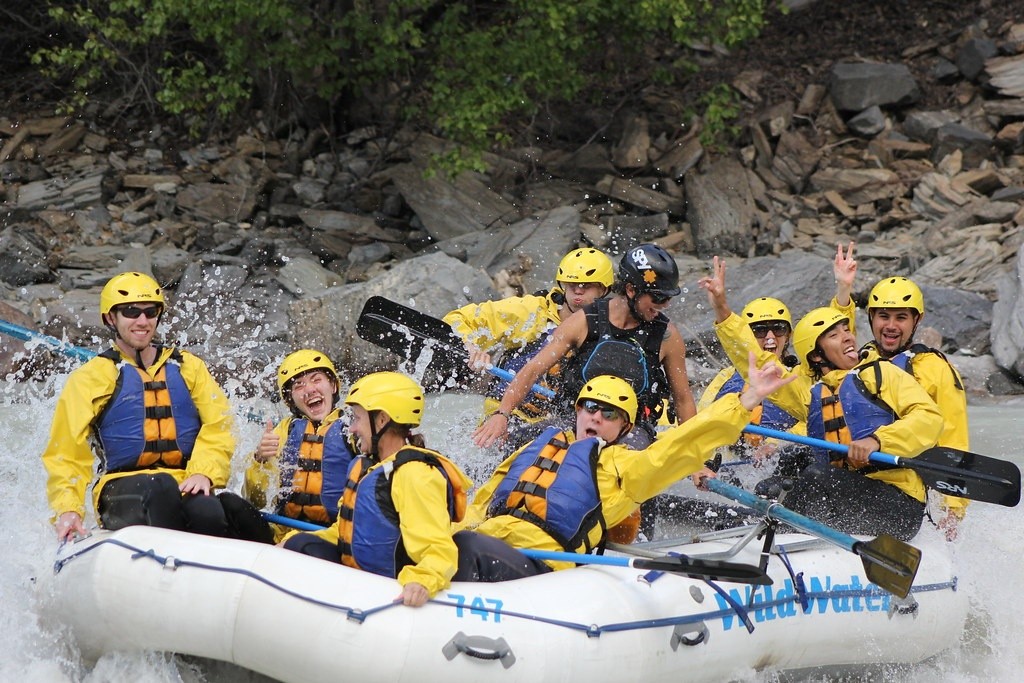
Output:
[253,508,775,588]
[0,321,283,427]
[353,292,925,600]
[742,419,1022,508]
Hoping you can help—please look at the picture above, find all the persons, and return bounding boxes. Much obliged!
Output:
[241,349,361,544]
[40,272,278,547]
[278,372,468,608]
[441,243,798,584]
[697,241,970,541]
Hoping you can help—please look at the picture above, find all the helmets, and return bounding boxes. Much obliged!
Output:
[345,371,424,428]
[867,277,924,315]
[100,271,165,325]
[278,349,340,407]
[617,244,681,296]
[792,307,849,369]
[741,297,793,330]
[555,248,615,287]
[575,375,638,431]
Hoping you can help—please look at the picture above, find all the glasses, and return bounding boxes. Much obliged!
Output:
[648,292,672,304]
[584,400,619,422]
[117,305,159,319]
[752,322,787,338]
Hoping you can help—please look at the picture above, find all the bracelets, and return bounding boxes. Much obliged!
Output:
[489,411,509,422]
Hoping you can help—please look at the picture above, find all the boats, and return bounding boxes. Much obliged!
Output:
[49,510,969,683]
